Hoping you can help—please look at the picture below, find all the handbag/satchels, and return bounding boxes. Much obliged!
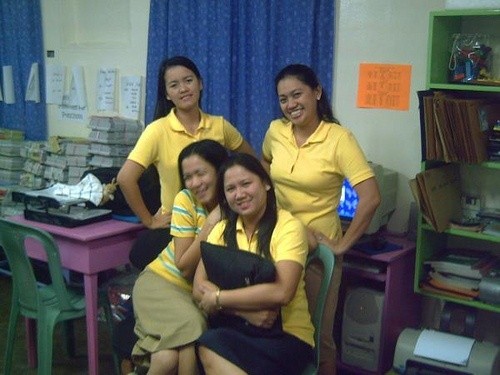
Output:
[81,167,145,216]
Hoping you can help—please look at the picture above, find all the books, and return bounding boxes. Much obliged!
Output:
[422,249,499,301]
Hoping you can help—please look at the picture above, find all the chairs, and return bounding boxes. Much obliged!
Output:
[0,218,123,375]
[303,244,334,375]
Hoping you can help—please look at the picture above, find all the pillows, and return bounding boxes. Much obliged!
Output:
[198,242,284,340]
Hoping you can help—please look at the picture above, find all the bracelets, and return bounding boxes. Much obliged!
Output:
[215,288,222,311]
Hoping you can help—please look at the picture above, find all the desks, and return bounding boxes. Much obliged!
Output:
[0,208,159,375]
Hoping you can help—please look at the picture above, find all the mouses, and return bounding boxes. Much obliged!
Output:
[368,237,389,252]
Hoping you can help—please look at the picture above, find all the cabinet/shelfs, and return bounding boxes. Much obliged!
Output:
[414,6,500,313]
[331,219,425,375]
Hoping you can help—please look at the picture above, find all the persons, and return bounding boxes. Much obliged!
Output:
[191,152,316,375]
[258,63,380,375]
[117,55,253,229]
[131,139,231,375]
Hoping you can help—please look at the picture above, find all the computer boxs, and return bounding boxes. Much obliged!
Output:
[340,281,385,372]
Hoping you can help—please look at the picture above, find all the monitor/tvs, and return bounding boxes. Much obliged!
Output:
[333,161,399,235]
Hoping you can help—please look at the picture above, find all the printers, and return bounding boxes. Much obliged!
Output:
[393,326,500,375]
